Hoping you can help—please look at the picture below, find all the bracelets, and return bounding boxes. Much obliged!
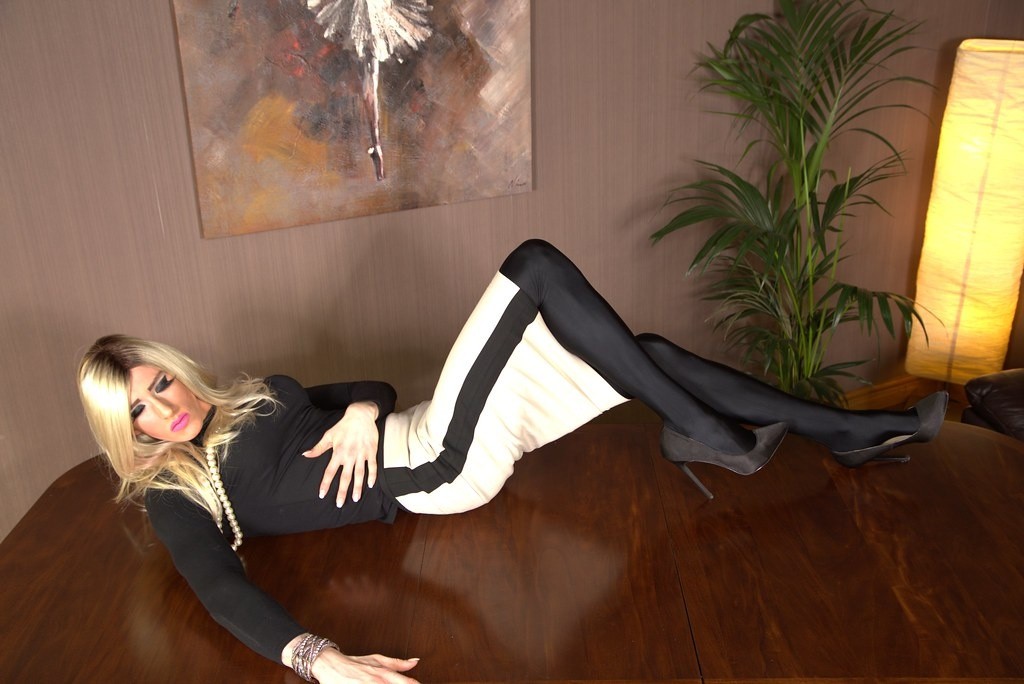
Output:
[291,634,340,683]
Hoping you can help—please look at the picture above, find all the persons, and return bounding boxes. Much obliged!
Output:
[79,239,945,683]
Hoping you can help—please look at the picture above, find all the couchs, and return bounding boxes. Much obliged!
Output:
[962,368,1023,438]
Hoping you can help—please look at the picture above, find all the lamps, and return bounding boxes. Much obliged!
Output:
[906,40,1023,388]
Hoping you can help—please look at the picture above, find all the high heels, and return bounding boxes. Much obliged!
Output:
[659,420,790,501]
[832,390,949,470]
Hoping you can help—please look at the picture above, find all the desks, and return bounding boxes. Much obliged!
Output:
[1,398,1024,683]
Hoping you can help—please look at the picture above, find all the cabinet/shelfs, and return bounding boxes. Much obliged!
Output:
[812,358,939,417]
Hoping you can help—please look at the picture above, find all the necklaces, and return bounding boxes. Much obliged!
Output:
[205,448,242,551]
